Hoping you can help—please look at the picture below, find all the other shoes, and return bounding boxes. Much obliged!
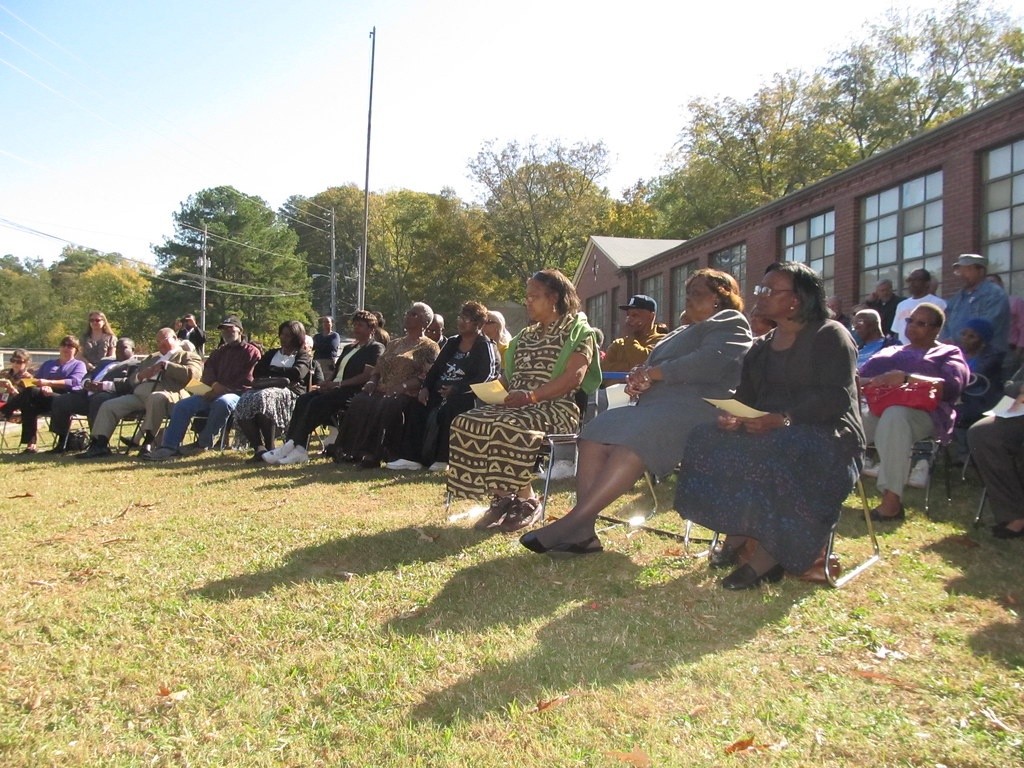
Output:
[908,460,930,489]
[990,521,1024,539]
[861,504,905,521]
[864,462,880,477]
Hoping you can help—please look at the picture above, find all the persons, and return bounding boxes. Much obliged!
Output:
[74,310,119,372]
[261,308,387,464]
[373,310,391,345]
[383,300,513,473]
[330,300,442,468]
[173,312,206,355]
[0,349,33,404]
[234,321,311,463]
[424,314,448,349]
[150,315,262,460]
[520,253,1024,594]
[304,315,340,382]
[45,336,139,454]
[440,268,603,530]
[0,334,88,454]
[483,310,513,372]
[75,328,203,459]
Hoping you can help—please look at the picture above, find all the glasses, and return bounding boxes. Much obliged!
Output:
[525,292,551,303]
[457,315,474,324]
[9,357,27,364]
[904,317,940,328]
[60,342,76,348]
[425,328,444,335]
[486,319,496,325]
[88,318,104,323]
[753,285,796,296]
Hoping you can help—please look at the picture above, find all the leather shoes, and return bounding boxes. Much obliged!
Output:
[722,562,784,590]
[711,546,738,570]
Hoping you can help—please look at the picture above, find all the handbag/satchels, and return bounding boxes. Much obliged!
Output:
[864,382,943,416]
[66,431,87,450]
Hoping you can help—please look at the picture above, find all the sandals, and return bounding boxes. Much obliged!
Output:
[553,536,604,554]
[519,531,551,554]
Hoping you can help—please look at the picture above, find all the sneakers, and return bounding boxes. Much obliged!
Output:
[0,400,450,474]
[499,493,543,533]
[474,492,513,530]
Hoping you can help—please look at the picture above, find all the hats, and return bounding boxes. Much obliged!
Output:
[216,317,243,330]
[618,295,657,312]
[951,254,988,268]
[967,319,993,341]
[905,268,930,283]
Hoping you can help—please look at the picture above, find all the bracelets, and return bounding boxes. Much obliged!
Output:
[641,370,651,382]
[529,391,537,401]
[338,381,342,387]
[366,381,374,384]
[903,372,909,383]
[779,412,791,427]
[401,382,408,389]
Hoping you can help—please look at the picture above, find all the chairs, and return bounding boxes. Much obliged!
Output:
[443,389,986,589]
[0,408,345,456]
